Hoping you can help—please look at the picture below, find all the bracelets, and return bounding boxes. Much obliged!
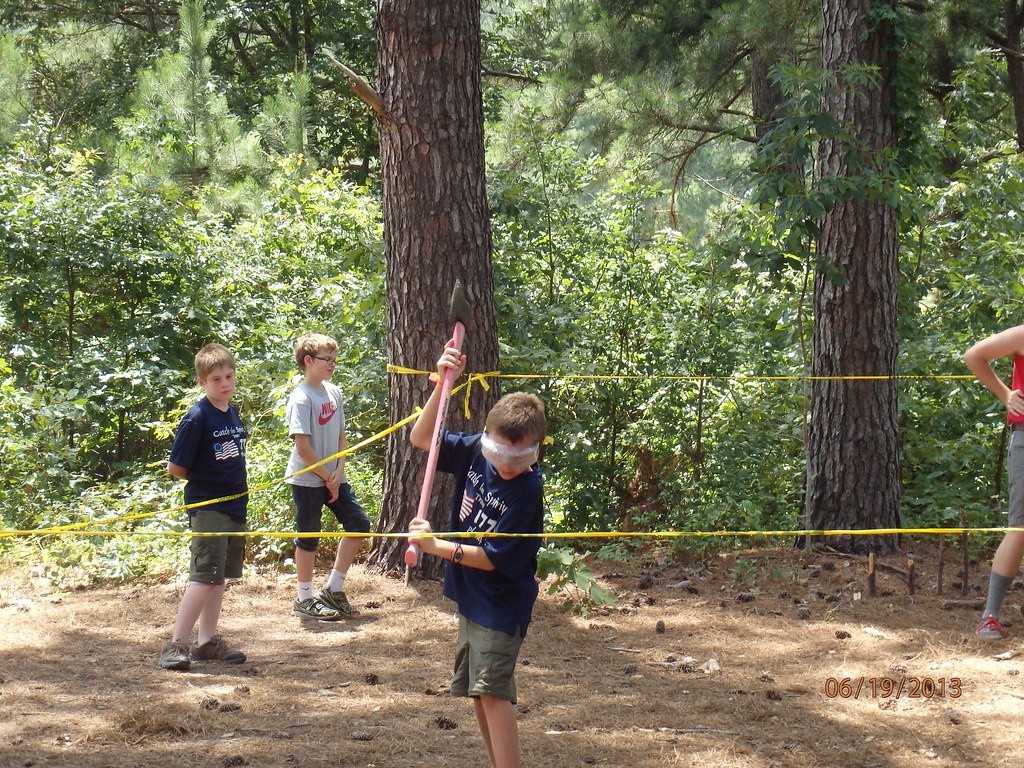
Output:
[451,542,463,563]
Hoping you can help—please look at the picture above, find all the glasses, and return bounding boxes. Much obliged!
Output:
[313,357,337,366]
[480,433,539,468]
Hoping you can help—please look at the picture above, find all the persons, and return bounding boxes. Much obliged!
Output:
[285,333,370,619]
[965,325,1024,640]
[407,338,547,768]
[158,343,249,669]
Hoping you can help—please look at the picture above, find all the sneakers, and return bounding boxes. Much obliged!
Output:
[158,642,190,670]
[314,588,360,616]
[975,612,1006,641]
[291,598,339,621]
[191,634,246,664]
